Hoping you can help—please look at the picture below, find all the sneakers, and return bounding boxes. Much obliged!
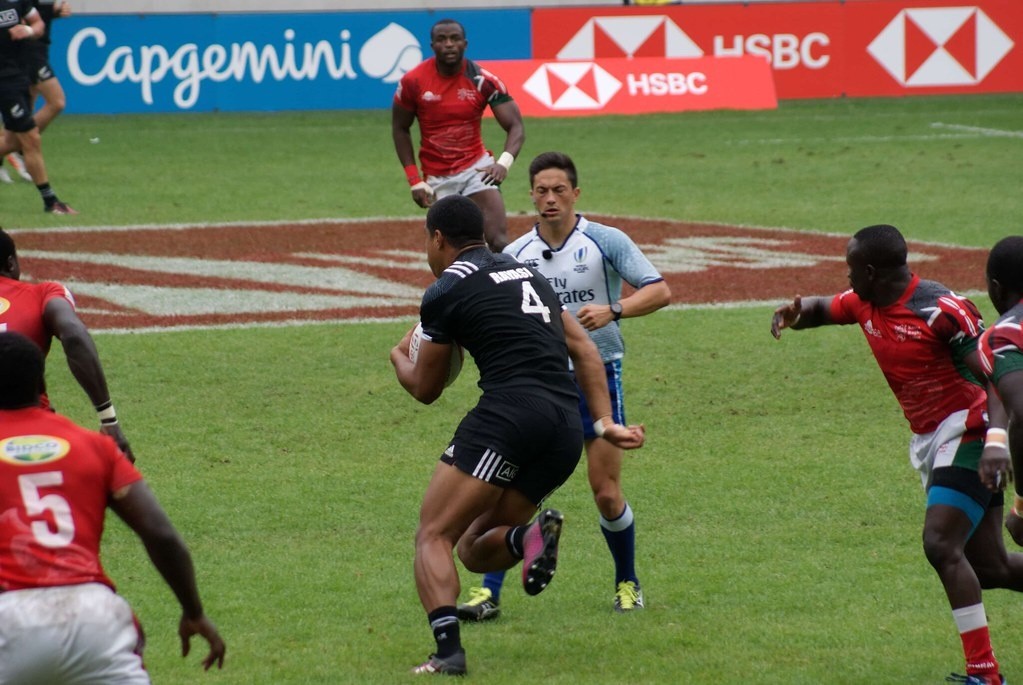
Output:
[613,577,644,612]
[522,509,563,595]
[409,648,467,676]
[456,587,500,621]
[945,672,1008,685]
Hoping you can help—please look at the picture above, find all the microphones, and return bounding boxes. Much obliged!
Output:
[543,250,552,259]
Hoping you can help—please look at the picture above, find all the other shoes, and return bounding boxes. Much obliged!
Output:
[0,166,13,182]
[45,202,79,215]
[7,152,33,182]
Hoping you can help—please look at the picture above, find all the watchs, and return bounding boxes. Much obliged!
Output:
[610,302,623,321]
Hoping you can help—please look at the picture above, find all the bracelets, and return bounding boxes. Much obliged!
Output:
[984,427,1007,448]
[25,24,34,35]
[594,416,615,436]
[790,314,800,326]
[497,151,514,170]
[96,399,118,426]
[1012,492,1023,517]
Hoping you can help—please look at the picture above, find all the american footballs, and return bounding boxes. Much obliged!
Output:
[407,321,465,390]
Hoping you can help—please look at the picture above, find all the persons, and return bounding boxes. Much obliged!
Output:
[390,195,646,675]
[456,151,671,620]
[0,331,226,685]
[0,230,136,464]
[0,1,78,212]
[392,19,526,253]
[771,224,1023,685]
[979,236,1023,545]
